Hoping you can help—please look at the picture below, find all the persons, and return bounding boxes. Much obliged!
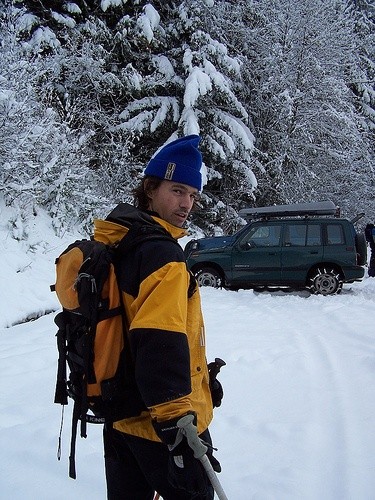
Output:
[367,220,375,278]
[48,134,227,500]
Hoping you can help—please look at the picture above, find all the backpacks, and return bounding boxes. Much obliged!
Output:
[48,225,184,420]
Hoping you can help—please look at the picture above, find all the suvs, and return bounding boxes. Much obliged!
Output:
[184,199,369,295]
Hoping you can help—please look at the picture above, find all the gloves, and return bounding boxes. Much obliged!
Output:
[206,362,223,409]
[151,409,222,473]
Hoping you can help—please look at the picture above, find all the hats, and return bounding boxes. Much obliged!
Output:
[144,134,202,191]
